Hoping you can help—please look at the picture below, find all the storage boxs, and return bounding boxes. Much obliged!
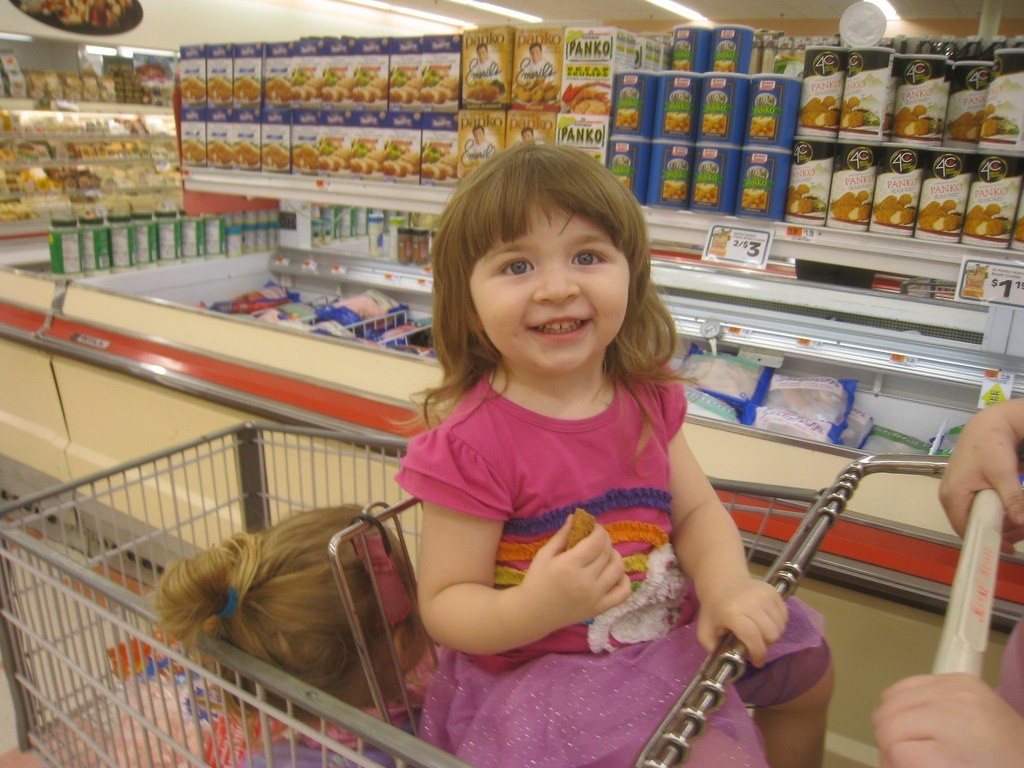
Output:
[180,22,672,196]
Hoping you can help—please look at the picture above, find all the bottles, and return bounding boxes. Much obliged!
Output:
[397,226,438,266]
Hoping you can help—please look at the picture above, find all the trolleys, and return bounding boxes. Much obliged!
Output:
[0,408,1007,767]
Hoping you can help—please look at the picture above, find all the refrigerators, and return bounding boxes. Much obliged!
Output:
[1,221,1024,627]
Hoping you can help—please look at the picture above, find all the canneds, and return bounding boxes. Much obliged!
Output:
[49,210,278,275]
[368,214,431,264]
[606,25,1024,252]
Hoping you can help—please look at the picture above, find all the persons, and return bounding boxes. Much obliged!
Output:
[393,136,833,768]
[873,395,1024,768]
[147,503,431,768]
